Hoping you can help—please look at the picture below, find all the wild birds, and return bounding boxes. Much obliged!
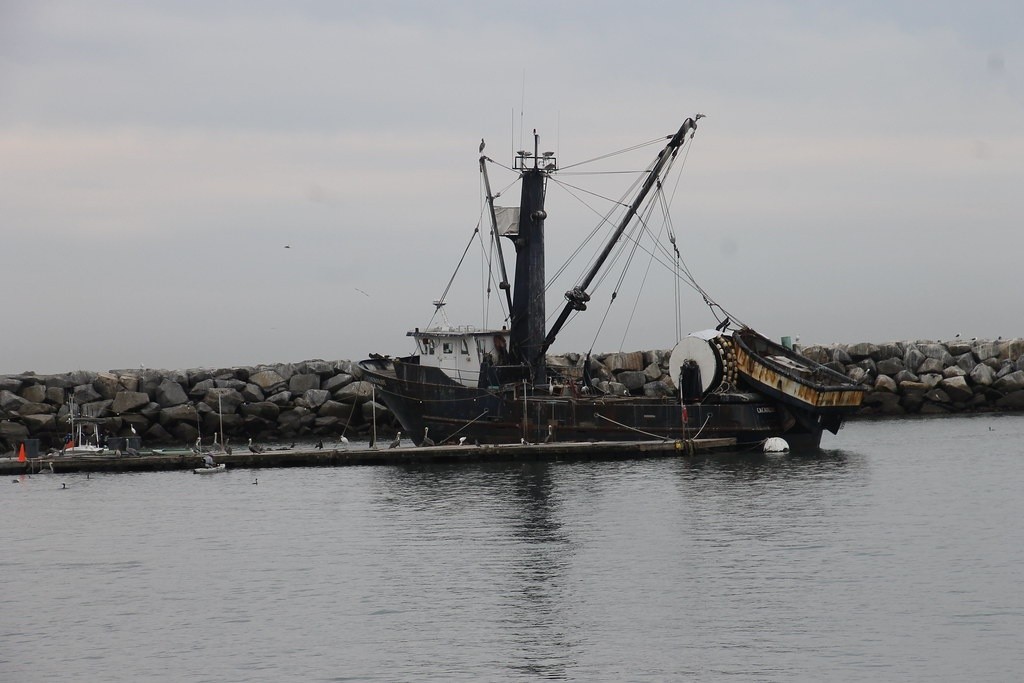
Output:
[23,422,557,491]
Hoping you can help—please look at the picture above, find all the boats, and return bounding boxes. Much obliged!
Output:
[153,449,194,455]
[37,393,122,459]
[194,463,225,474]
[353,111,827,449]
[731,326,868,414]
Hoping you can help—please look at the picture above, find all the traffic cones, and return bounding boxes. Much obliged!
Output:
[17,444,27,461]
